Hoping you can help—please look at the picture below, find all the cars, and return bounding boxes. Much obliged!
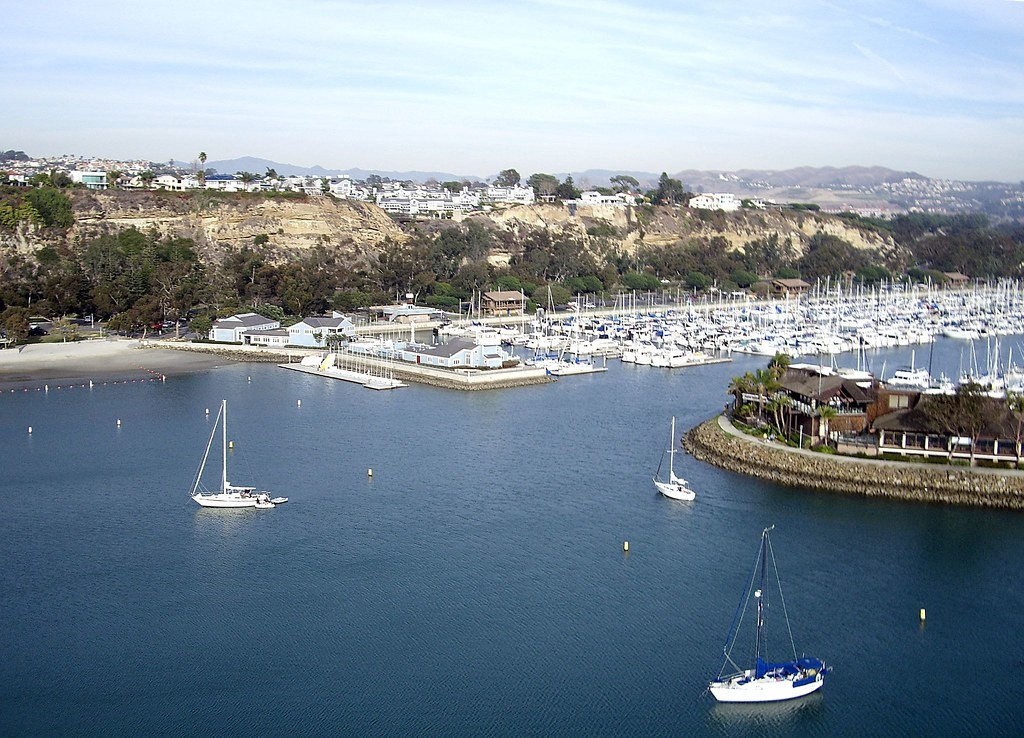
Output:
[150,317,187,329]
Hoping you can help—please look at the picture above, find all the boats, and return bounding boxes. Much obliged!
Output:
[255,500,276,508]
[271,496,289,504]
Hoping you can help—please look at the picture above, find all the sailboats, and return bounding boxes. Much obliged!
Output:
[652,416,697,501]
[448,268,1024,401]
[701,525,833,704]
[183,397,272,509]
